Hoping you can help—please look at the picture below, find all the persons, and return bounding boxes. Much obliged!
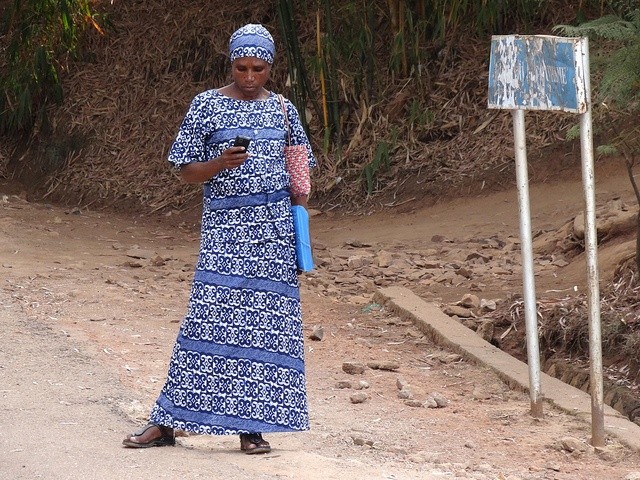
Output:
[122,24,316,454]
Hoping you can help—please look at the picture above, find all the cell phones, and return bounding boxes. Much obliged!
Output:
[234,136,252,153]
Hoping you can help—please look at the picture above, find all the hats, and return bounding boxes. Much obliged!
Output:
[229,24,276,65]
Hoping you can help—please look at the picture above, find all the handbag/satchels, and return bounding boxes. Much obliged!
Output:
[283,143,311,197]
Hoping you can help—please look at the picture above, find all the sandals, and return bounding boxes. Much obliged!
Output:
[122,421,175,448]
[240,432,271,454]
